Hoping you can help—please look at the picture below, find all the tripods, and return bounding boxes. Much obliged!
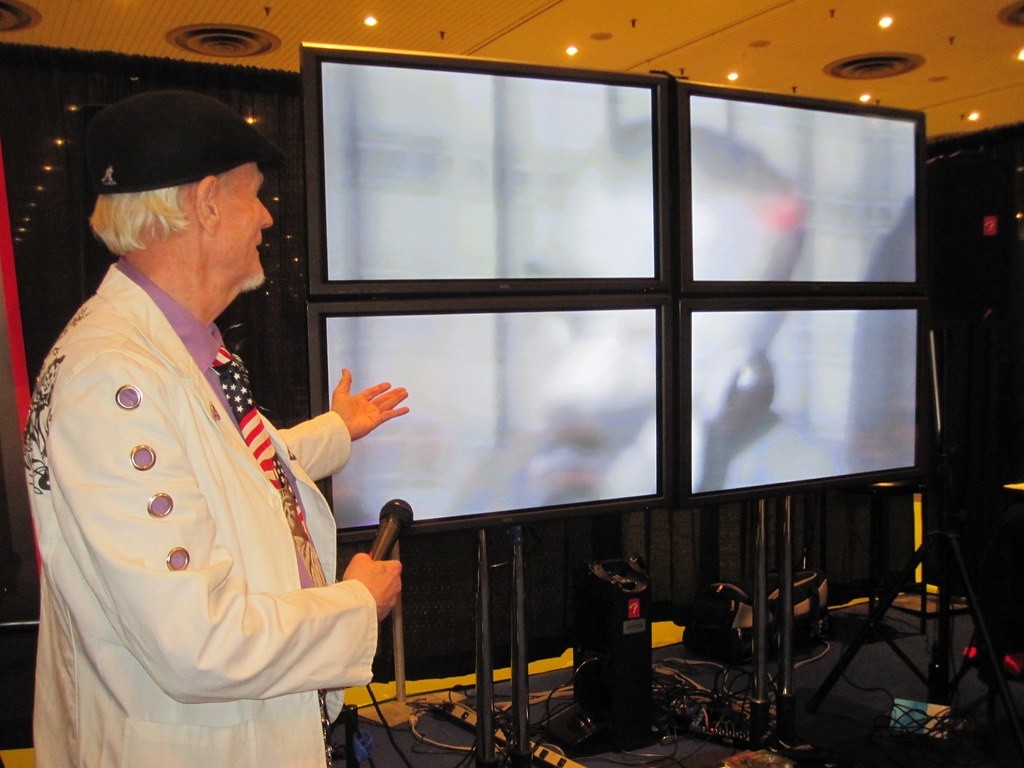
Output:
[797,314,1024,759]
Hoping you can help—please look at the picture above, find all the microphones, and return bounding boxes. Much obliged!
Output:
[369,499,414,562]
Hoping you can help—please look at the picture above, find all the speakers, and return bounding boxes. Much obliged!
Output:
[925,136,1017,313]
[76,101,124,299]
[570,548,654,748]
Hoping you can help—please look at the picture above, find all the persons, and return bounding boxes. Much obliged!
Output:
[22,88,411,768]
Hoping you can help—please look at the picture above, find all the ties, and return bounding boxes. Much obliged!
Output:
[210,347,329,588]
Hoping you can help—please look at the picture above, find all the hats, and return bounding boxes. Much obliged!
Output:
[85,91,287,193]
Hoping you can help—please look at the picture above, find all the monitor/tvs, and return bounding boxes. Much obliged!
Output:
[299,40,675,295]
[306,295,677,545]
[674,295,930,513]
[676,75,926,297]
